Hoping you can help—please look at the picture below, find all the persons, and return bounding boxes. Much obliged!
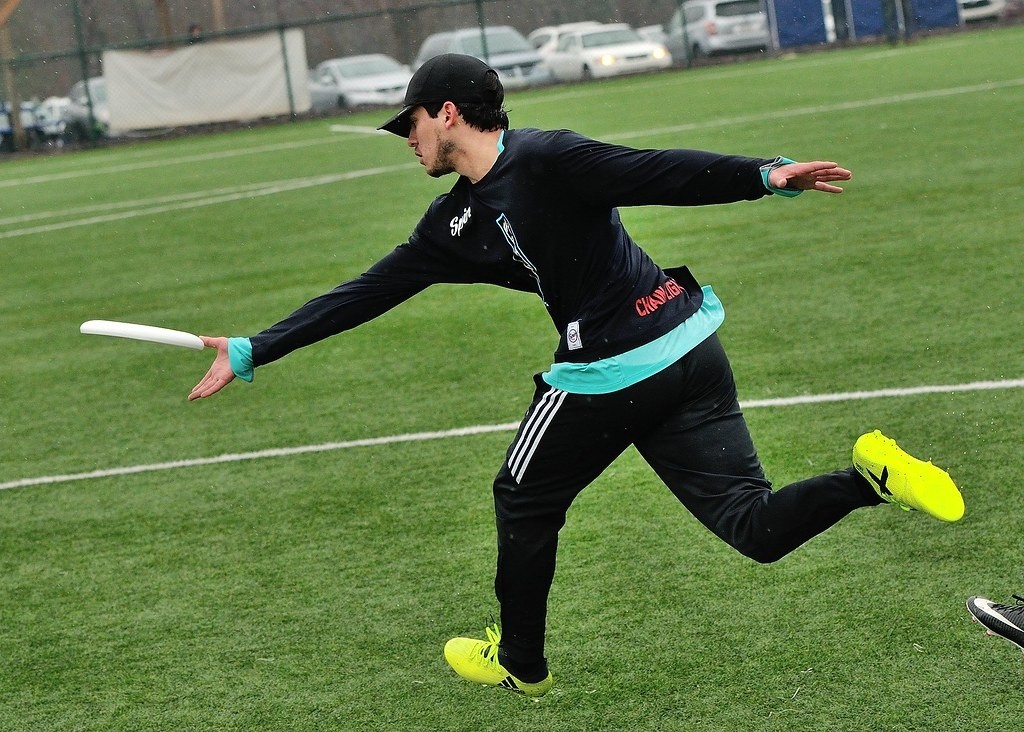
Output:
[186,52,964,696]
[965,593,1024,651]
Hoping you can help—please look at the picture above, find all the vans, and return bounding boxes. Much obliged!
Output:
[670,0,772,66]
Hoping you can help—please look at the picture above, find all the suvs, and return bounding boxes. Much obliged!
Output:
[416,26,552,95]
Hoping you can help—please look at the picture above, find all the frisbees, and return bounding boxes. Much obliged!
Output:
[79,316,206,351]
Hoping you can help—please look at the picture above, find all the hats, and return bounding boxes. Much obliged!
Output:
[377,54,505,138]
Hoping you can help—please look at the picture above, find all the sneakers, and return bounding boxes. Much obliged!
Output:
[444,613,554,699]
[966,592,1024,651]
[853,430,966,522]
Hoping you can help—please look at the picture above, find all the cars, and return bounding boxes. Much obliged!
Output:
[69,75,175,142]
[309,52,415,113]
[529,19,673,84]
